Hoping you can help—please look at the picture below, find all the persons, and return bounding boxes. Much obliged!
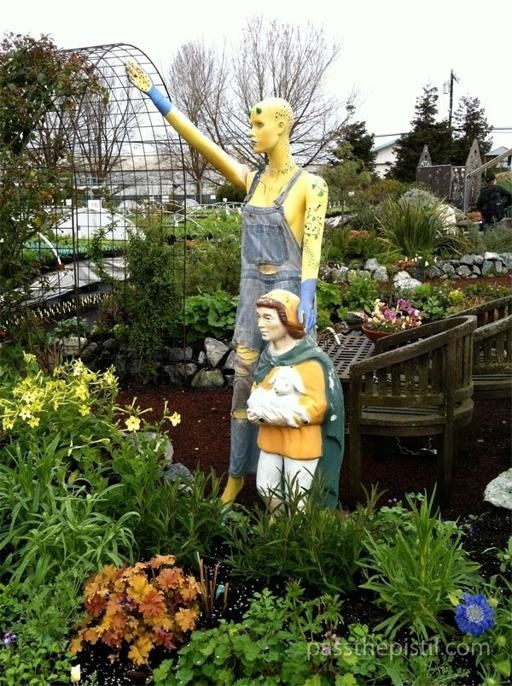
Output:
[246,288,346,523]
[476,173,512,231]
[123,61,330,530]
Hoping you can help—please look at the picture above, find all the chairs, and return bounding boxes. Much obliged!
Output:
[339,290,512,508]
